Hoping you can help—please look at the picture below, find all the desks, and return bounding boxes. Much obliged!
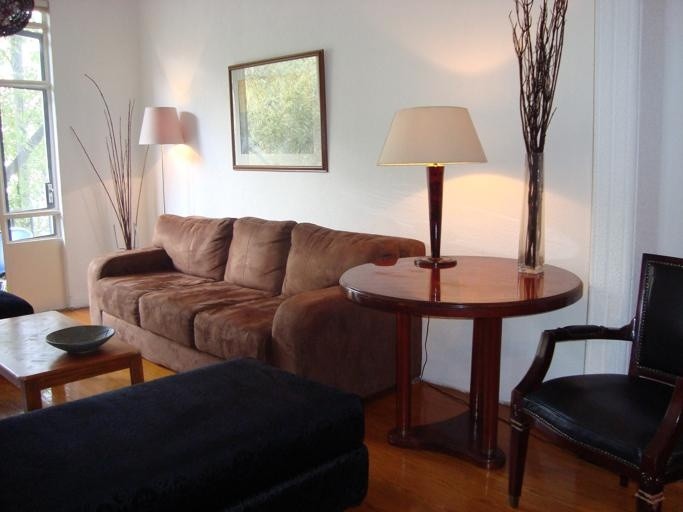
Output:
[340,255,584,470]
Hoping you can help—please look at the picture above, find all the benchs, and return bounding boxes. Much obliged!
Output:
[0,357,367,511]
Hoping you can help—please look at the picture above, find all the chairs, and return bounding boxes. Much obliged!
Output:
[511,254,682,507]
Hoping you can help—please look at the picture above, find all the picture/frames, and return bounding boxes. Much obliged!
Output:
[228,50,329,171]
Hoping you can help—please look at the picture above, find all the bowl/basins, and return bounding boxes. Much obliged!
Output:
[45,325,115,355]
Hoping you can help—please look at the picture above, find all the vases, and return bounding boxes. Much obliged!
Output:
[519,155,544,276]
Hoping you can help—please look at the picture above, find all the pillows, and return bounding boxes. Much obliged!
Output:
[151,214,400,297]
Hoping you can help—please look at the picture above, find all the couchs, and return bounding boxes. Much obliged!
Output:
[87,234,426,399]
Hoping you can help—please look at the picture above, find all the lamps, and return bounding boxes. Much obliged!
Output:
[139,108,184,213]
[376,108,489,270]
